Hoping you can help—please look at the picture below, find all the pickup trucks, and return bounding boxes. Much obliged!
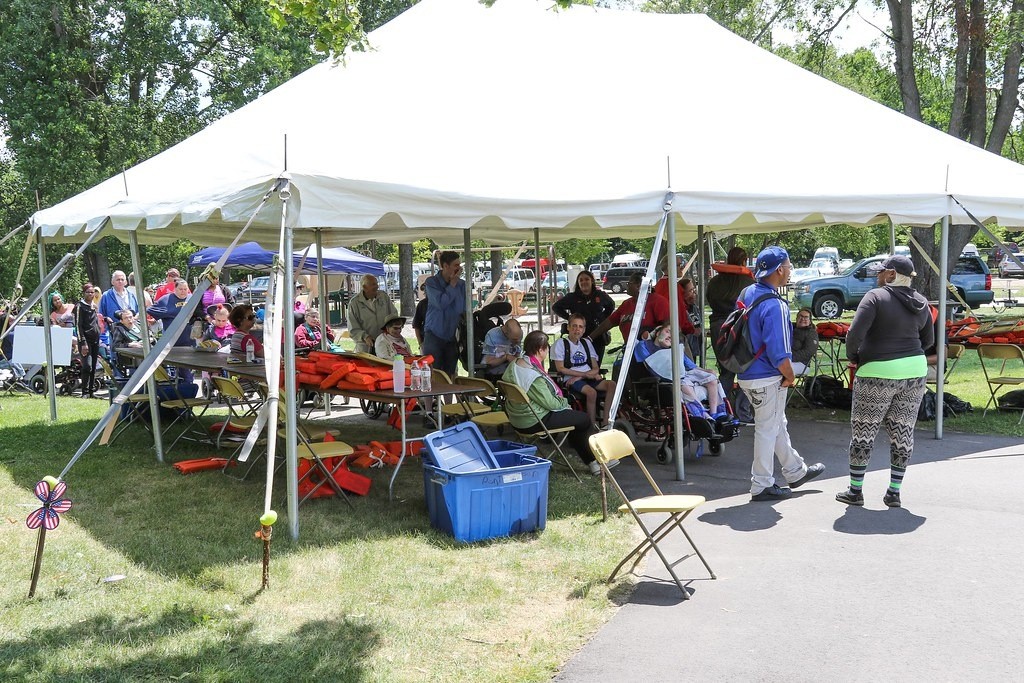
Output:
[792,251,994,319]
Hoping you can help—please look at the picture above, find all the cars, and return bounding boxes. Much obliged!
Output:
[588,253,650,279]
[242,276,276,303]
[542,272,568,288]
[998,253,1024,278]
[227,281,247,301]
[893,246,911,255]
[959,244,979,258]
[788,247,855,290]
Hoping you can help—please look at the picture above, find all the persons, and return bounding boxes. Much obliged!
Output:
[835,255,934,506]
[735,247,825,501]
[48,247,816,477]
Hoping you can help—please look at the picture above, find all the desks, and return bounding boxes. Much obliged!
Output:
[116,346,486,505]
[809,335,1024,411]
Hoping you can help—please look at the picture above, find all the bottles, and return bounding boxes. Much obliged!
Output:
[410,360,421,391]
[421,361,431,392]
[392,354,405,393]
[246,339,254,362]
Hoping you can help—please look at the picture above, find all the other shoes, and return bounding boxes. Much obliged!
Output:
[590,458,620,475]
[90,394,102,400]
[81,395,87,399]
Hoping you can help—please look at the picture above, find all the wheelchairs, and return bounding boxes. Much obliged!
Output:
[294,312,350,408]
[608,326,747,465]
[454,302,608,428]
[360,338,445,420]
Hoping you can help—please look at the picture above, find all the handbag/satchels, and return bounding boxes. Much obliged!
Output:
[190,317,202,339]
[602,330,612,346]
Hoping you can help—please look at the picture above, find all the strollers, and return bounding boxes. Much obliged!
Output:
[201,303,255,404]
[31,321,105,396]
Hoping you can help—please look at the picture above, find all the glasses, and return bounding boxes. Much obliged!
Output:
[677,263,682,268]
[391,326,402,330]
[243,315,255,321]
[798,315,810,320]
[687,288,696,293]
[780,263,794,270]
[85,291,94,294]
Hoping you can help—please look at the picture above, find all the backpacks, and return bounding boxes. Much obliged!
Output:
[716,286,781,374]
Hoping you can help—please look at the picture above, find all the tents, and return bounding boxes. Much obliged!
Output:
[28,0,1024,545]
[186,242,384,324]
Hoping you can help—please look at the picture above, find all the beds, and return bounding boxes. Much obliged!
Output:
[257,381,340,494]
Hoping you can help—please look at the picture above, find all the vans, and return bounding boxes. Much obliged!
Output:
[986,242,1020,269]
[602,267,648,294]
[473,269,535,293]
[344,263,439,299]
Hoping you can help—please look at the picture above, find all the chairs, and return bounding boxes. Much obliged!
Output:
[210,375,286,483]
[496,380,583,483]
[150,364,217,454]
[925,344,965,418]
[977,343,1024,424]
[96,357,160,447]
[785,352,815,411]
[588,428,717,600]
[453,376,520,442]
[432,368,491,430]
[277,400,356,513]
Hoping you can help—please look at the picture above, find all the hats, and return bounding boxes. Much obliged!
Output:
[755,246,788,279]
[381,313,406,329]
[294,281,305,288]
[870,254,917,278]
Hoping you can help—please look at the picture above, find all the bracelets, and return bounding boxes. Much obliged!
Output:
[587,336,593,342]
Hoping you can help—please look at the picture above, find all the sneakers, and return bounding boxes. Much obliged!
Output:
[836,491,864,504]
[789,463,825,488]
[752,484,792,500]
[883,495,901,507]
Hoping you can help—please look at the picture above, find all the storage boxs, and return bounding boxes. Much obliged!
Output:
[422,421,552,543]
[106,377,199,422]
[419,439,538,464]
